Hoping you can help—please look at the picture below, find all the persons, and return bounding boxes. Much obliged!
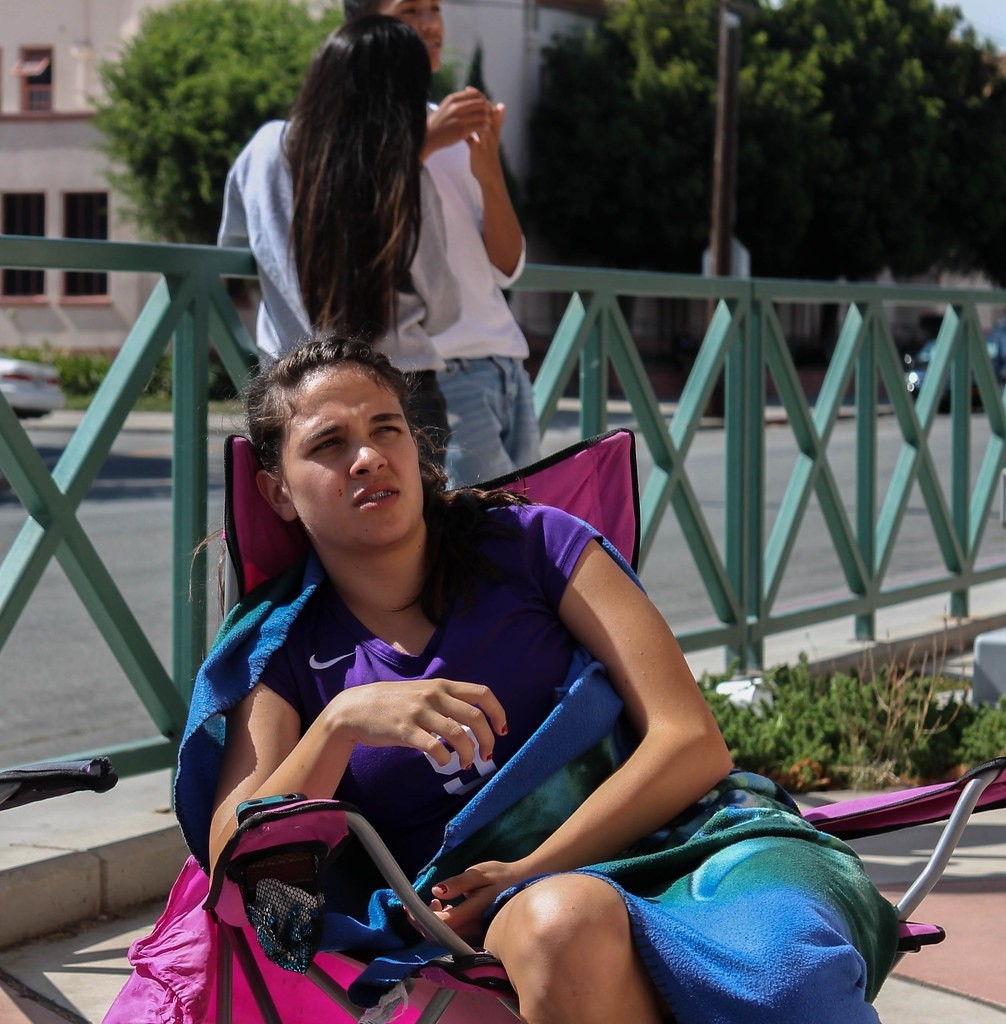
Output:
[217,13,464,485]
[342,1,541,490]
[169,332,906,1024]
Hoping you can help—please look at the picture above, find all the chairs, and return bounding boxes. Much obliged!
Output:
[188,428,1005,1024]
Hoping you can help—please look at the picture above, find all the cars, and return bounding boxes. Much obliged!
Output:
[0,357,63,419]
[903,329,1006,410]
